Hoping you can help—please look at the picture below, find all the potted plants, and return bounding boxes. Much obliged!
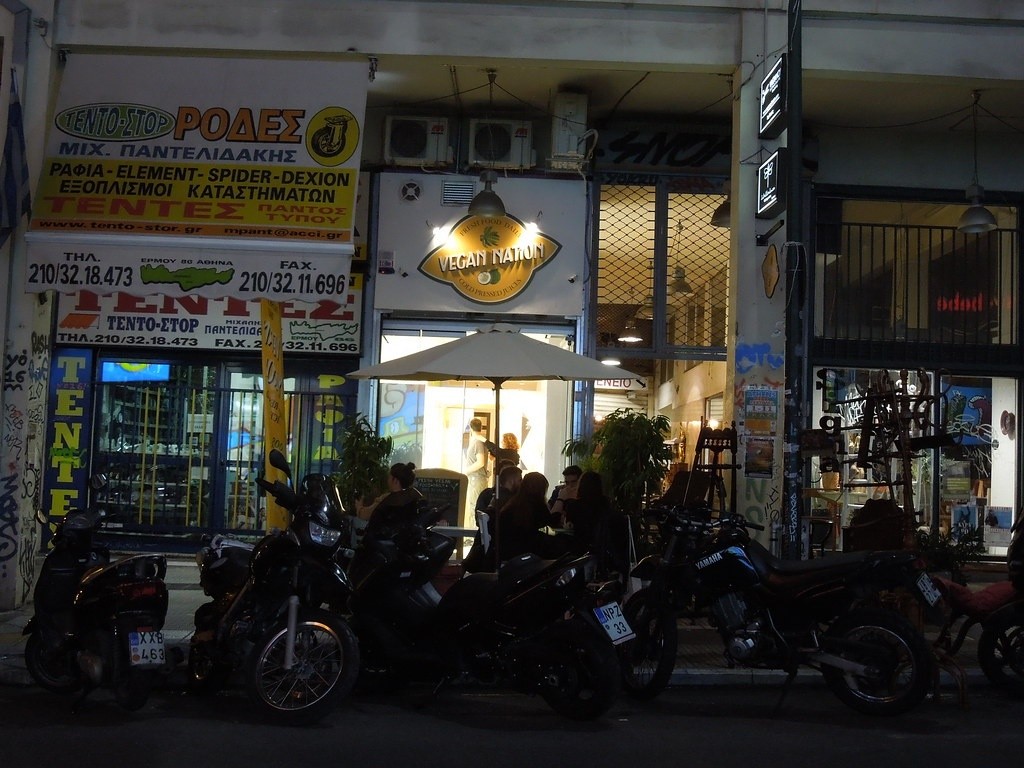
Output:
[329,413,391,546]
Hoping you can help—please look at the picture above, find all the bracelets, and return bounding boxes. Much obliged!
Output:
[556,497,565,502]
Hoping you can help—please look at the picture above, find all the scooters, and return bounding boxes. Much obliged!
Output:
[357,506,639,724]
[20,510,167,723]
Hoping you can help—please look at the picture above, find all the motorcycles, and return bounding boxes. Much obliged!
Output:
[646,491,935,720]
[192,449,352,720]
[920,519,1024,703]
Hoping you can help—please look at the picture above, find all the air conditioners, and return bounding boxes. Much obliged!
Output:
[550,97,588,170]
[467,118,541,171]
[383,116,453,168]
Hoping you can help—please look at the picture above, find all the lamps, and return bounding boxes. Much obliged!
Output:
[670,223,695,293]
[634,262,656,320]
[957,89,999,234]
[618,291,643,343]
[467,74,506,218]
[368,56,378,82]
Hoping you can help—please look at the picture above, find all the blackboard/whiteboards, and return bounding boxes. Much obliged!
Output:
[410,468,467,562]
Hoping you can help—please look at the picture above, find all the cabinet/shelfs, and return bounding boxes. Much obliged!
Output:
[103,364,183,442]
[840,393,921,547]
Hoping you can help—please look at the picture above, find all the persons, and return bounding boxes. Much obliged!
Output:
[460,419,487,547]
[355,461,417,521]
[483,434,520,470]
[461,466,610,573]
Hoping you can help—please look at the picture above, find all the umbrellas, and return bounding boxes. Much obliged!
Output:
[344,323,642,566]
